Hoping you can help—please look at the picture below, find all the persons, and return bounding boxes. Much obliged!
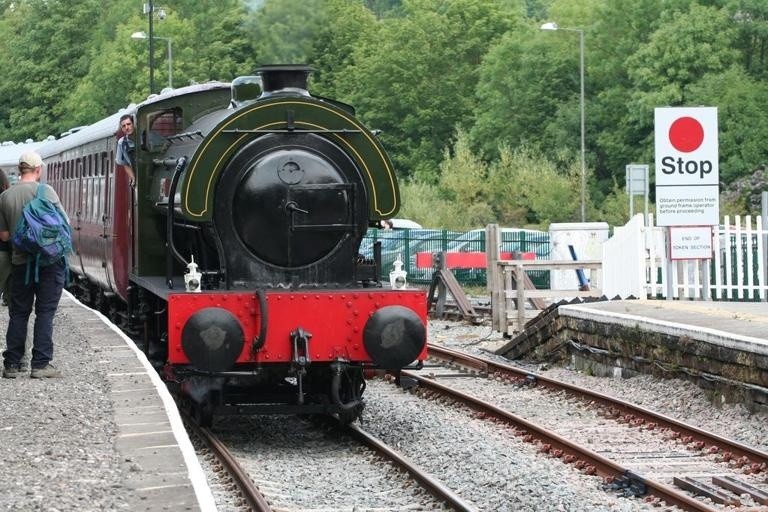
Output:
[0,151,73,381]
[1,169,13,307]
[113,113,140,188]
[382,220,395,232]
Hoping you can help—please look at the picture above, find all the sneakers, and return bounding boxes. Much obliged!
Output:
[28,363,65,380]
[1,363,31,379]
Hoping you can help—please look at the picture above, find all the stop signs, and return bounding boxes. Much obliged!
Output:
[652,105,720,187]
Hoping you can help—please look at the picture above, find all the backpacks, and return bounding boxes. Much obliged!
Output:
[12,183,72,266]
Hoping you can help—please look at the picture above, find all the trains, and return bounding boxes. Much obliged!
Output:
[0,59,424,434]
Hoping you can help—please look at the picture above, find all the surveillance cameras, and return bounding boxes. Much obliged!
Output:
[158,11,166,20]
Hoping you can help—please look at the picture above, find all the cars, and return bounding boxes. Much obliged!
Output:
[359,221,550,289]
[625,213,758,300]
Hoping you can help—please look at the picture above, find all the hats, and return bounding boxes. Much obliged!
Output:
[16,151,46,169]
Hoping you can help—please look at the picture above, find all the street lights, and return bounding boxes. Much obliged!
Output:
[538,20,587,224]
[130,31,173,88]
